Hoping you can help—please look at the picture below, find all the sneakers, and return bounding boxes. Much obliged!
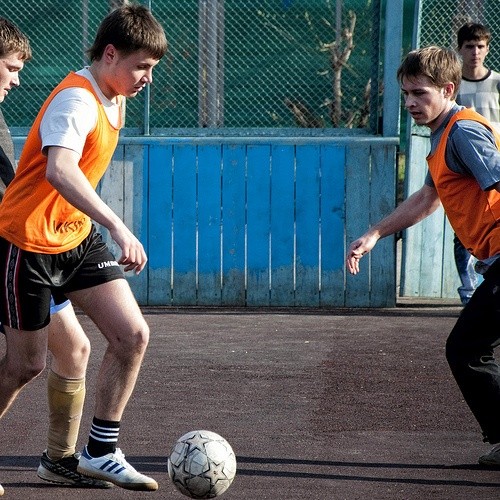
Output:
[76,445,158,491]
[37,448,115,489]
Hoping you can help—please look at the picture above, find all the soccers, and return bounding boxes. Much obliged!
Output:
[166,429,238,499]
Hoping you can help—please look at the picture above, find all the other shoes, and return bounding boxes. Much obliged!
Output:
[478,445,500,466]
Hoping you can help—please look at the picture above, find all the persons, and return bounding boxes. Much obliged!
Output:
[345,46,500,469]
[455,24,500,305]
[0,4,162,494]
[0,17,116,489]
